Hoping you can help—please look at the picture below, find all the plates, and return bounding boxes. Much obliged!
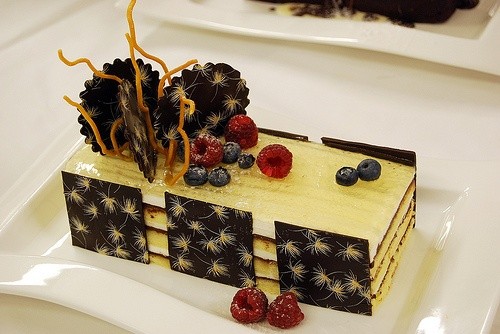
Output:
[1,0,500,334]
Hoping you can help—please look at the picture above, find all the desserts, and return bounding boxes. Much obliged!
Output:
[57,0,419,316]
